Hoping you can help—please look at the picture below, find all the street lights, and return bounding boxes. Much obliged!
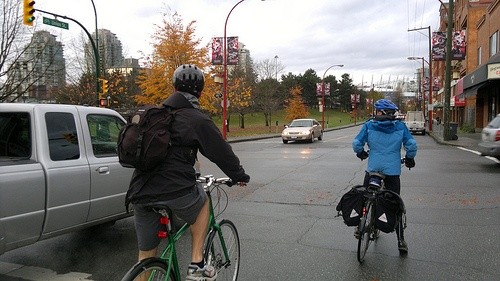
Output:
[223,0,265,142]
[354,82,368,126]
[408,26,433,130]
[407,56,425,118]
[318,64,345,133]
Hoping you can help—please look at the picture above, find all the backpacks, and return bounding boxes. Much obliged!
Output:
[118,104,204,168]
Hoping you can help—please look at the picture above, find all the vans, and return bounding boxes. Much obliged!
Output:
[0,103,138,253]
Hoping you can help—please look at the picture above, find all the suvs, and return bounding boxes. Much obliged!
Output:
[477,114,500,162]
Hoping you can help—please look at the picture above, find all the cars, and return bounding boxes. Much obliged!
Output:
[395,114,406,120]
[282,118,323,144]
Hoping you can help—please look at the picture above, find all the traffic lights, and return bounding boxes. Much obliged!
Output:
[22,0,35,26]
[100,98,108,106]
[100,79,109,93]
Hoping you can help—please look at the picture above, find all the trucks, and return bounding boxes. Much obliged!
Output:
[406,111,426,135]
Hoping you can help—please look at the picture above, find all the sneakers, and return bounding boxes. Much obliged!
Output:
[354,230,362,239]
[185,264,218,281]
[397,240,408,252]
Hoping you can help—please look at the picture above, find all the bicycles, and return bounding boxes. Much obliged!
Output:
[121,171,247,281]
[354,150,411,263]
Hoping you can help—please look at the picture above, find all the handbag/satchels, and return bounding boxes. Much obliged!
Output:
[335,185,407,233]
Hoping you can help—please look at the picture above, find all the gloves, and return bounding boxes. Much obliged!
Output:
[357,148,368,159]
[405,155,415,168]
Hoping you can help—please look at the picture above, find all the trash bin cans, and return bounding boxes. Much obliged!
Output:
[449,122,458,140]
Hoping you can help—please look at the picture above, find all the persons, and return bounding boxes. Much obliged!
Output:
[127,64,249,281]
[352,99,417,253]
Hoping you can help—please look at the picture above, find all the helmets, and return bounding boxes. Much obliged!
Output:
[173,64,205,97]
[373,99,399,110]
